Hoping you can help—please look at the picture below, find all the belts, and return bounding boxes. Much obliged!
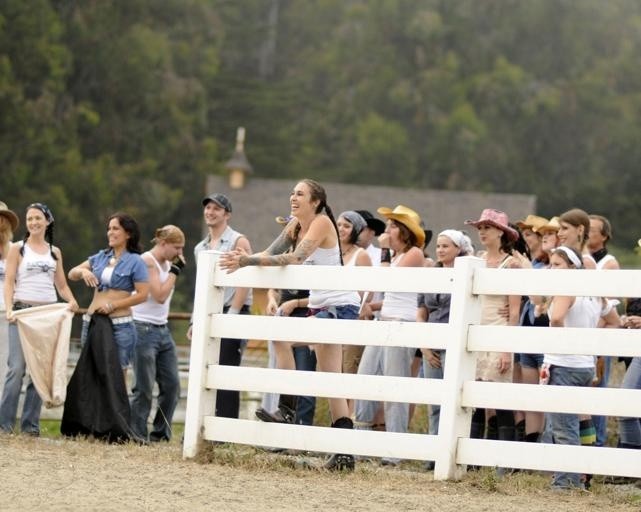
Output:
[14,302,32,309]
[82,313,132,325]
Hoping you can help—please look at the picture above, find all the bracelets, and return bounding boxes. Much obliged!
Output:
[105,302,114,313]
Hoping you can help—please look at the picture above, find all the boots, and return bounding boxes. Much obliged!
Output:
[467,422,532,480]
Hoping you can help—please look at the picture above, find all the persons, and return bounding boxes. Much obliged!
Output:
[125,222,187,441]
[218,176,357,472]
[266,206,641,489]
[69,210,150,376]
[186,192,253,446]
[1,201,79,438]
[1,198,15,398]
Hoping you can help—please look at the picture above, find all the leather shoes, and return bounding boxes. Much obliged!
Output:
[256,407,292,424]
[318,454,355,470]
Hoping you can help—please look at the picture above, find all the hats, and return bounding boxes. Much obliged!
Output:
[463,208,561,245]
[0,201,20,232]
[202,193,232,212]
[356,204,432,249]
